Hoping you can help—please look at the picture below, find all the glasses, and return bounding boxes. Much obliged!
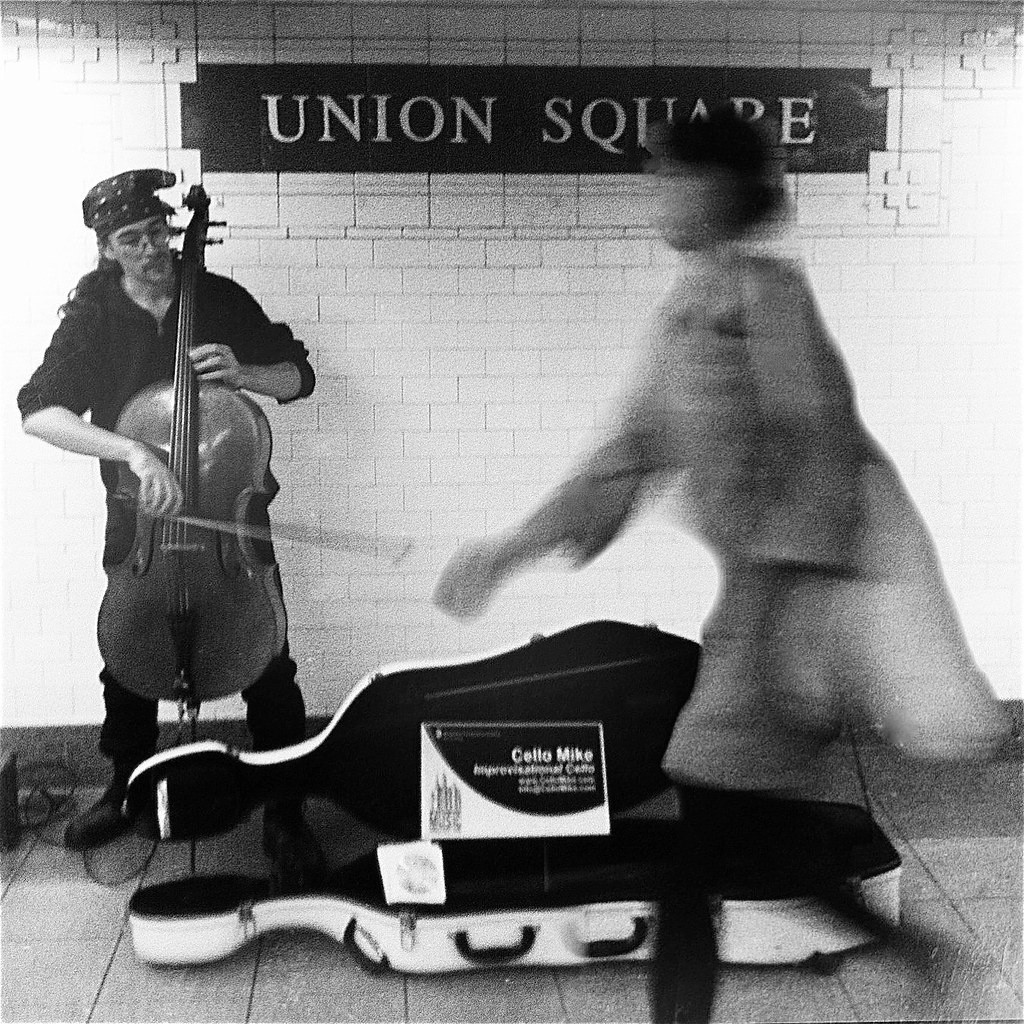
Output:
[108,231,171,249]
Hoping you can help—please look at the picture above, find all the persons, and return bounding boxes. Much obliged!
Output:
[18,169,328,879]
[435,109,1009,1024]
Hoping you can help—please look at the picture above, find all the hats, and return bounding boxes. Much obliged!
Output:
[82,169,176,235]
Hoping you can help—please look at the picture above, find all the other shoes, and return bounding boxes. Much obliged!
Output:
[64,771,130,850]
[262,810,327,877]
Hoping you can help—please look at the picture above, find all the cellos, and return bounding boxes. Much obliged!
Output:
[92,182,289,879]
